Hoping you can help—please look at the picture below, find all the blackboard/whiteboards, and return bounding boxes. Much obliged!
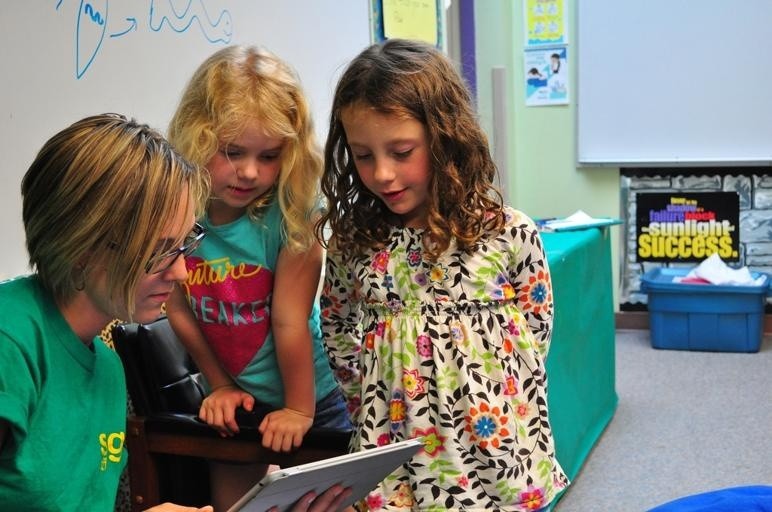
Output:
[574,0,771,168]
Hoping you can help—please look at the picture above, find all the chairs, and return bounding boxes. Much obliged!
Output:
[111,309,354,512]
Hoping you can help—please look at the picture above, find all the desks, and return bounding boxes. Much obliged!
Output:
[536,217,619,511]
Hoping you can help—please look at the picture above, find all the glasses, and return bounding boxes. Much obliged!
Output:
[107,222,206,274]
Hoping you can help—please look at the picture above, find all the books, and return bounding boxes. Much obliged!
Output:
[540,208,622,231]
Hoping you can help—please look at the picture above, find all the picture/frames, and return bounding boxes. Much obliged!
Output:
[523,46,569,108]
[522,1,569,49]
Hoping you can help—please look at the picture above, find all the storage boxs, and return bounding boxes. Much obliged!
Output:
[640,264,767,353]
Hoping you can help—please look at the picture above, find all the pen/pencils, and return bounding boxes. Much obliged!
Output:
[135,496,159,509]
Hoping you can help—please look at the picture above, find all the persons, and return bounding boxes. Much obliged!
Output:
[528,68,547,80]
[321,38,572,512]
[0,112,355,511]
[540,53,568,93]
[164,45,355,510]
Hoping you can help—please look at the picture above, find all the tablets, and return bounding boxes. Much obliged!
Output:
[226,435,428,512]
